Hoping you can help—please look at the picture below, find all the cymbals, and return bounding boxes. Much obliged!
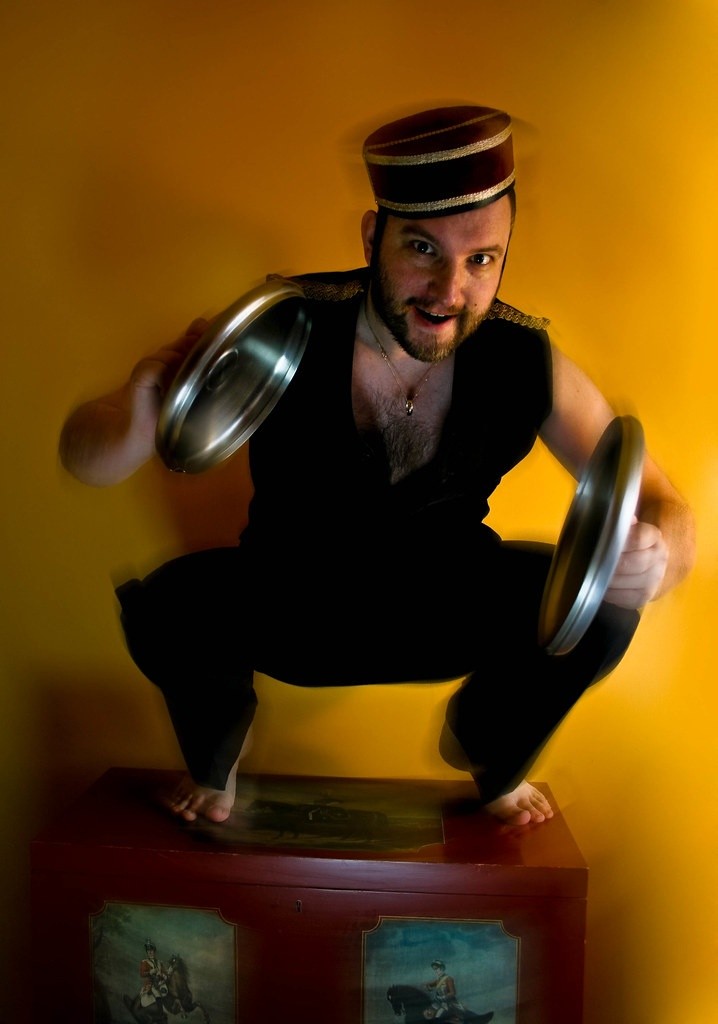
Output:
[535,413,645,658]
[153,272,315,475]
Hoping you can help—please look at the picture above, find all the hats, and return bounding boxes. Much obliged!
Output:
[363,105,515,220]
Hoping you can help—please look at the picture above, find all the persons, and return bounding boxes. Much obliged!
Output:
[61,104,698,826]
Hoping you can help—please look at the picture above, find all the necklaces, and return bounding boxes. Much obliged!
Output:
[361,309,449,415]
